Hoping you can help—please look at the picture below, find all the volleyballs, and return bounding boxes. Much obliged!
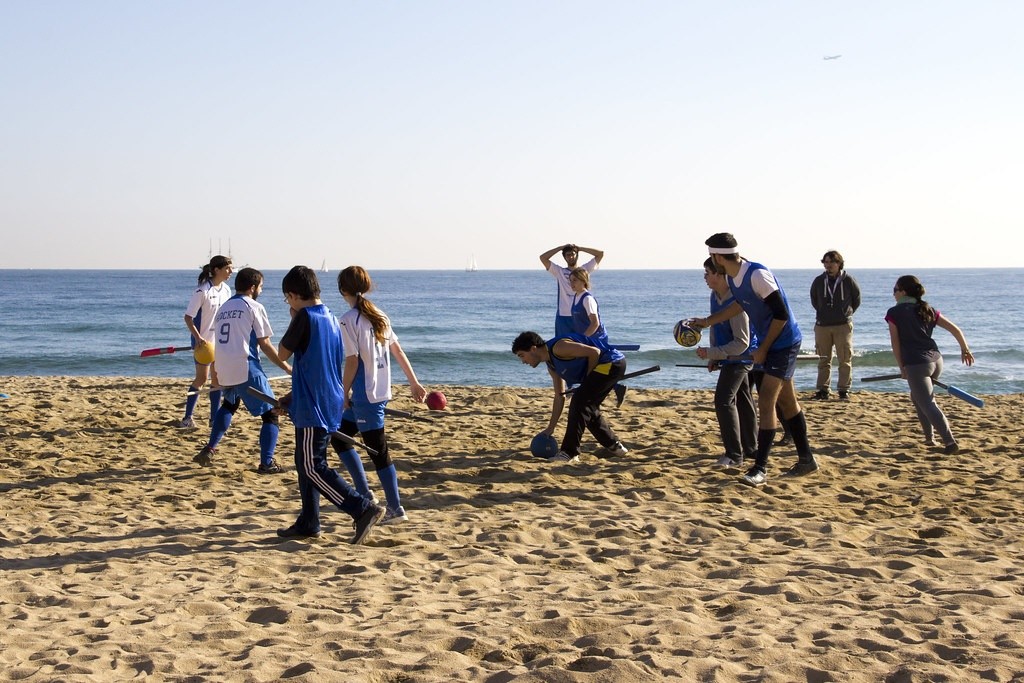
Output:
[531,434,558,458]
[426,391,446,411]
[673,318,701,347]
[194,342,215,364]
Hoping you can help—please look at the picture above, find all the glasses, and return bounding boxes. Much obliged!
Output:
[894,288,906,293]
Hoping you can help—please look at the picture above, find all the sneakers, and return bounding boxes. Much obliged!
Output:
[809,390,829,401]
[258,460,288,474]
[365,489,380,505]
[741,465,767,486]
[715,454,742,467]
[839,391,849,399]
[606,441,630,457]
[779,457,820,478]
[617,385,627,408]
[194,445,215,468]
[774,435,794,446]
[379,505,408,526]
[355,502,386,545]
[179,417,198,430]
[209,419,215,427]
[548,449,578,464]
[277,519,324,539]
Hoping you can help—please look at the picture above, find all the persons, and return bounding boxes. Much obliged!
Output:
[686,232,817,483]
[181,256,233,425]
[539,244,604,338]
[695,258,797,468]
[566,268,627,408]
[194,267,287,475]
[276,266,386,545]
[883,275,975,453]
[510,333,630,461]
[809,251,862,400]
[331,266,427,525]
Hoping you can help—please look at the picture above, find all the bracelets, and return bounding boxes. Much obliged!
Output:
[705,318,710,327]
[961,350,969,355]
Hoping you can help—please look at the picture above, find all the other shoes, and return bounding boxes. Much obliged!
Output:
[944,444,959,451]
